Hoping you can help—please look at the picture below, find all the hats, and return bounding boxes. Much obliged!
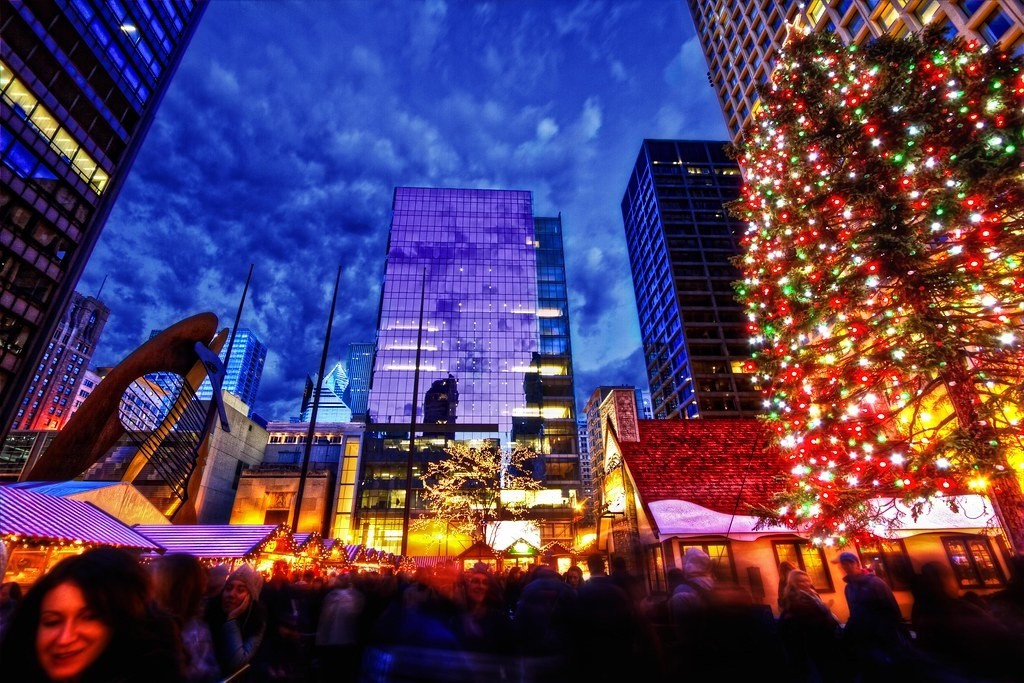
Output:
[831,552,858,564]
[223,563,259,600]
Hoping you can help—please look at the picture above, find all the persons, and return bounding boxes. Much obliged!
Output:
[0,544,1024,683]
